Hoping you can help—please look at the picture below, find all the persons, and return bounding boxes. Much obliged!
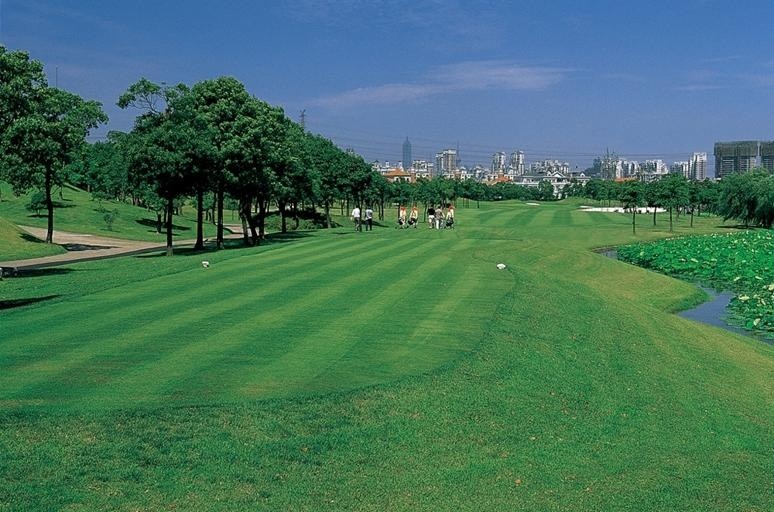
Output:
[398,206,418,229]
[427,204,455,230]
[364,206,373,231]
[352,204,360,231]
